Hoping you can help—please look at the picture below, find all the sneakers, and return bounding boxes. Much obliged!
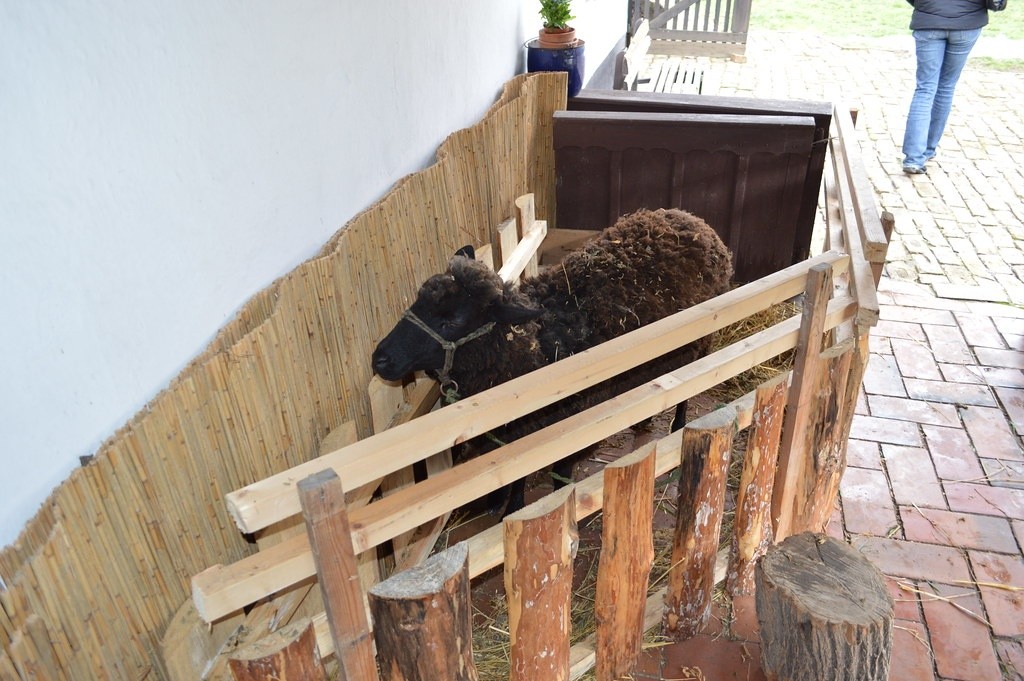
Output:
[929,152,937,161]
[902,163,928,174]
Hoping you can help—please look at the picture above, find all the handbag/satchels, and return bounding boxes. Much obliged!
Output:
[985,0,1007,11]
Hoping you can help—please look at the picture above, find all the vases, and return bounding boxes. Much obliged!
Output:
[524,28,586,98]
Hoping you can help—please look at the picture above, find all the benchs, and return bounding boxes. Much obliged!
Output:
[623,19,713,95]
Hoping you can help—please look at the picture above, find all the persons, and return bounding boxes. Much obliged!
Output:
[900,1,1006,175]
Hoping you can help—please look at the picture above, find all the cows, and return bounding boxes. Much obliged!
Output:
[371,206,736,525]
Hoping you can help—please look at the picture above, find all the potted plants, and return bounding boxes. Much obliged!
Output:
[539,0,576,33]
